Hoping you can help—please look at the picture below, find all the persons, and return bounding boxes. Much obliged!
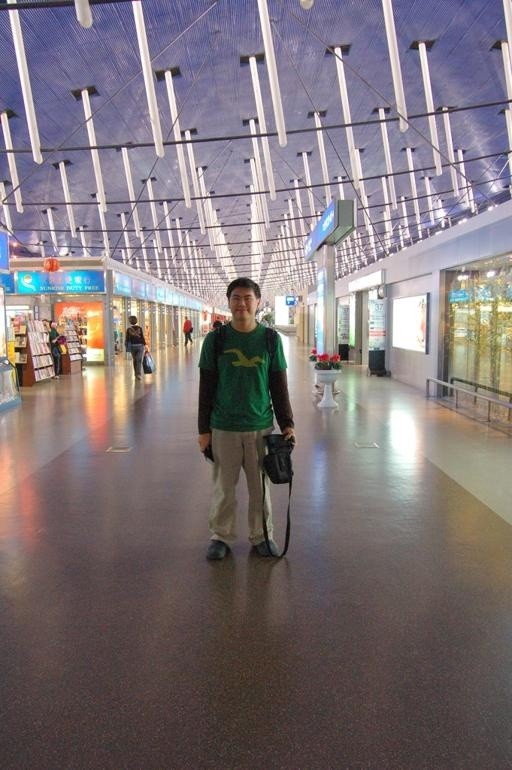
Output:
[195,275,297,562]
[212,316,223,329]
[125,315,151,381]
[182,316,193,346]
[260,318,269,328]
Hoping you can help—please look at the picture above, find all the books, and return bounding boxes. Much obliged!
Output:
[13,316,83,383]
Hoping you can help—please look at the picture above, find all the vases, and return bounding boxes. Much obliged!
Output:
[311,367,342,409]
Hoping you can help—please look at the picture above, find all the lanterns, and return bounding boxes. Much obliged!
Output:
[44,257,60,272]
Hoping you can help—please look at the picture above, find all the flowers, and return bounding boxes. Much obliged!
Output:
[309,348,344,369]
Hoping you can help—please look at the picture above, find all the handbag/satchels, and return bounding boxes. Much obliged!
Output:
[262,433,295,483]
[126,341,130,352]
[143,352,156,374]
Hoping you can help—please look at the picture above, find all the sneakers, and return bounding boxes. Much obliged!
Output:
[257,538,278,556]
[137,375,142,380]
[205,539,228,560]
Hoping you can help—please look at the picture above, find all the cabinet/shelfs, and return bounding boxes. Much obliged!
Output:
[10,319,83,386]
